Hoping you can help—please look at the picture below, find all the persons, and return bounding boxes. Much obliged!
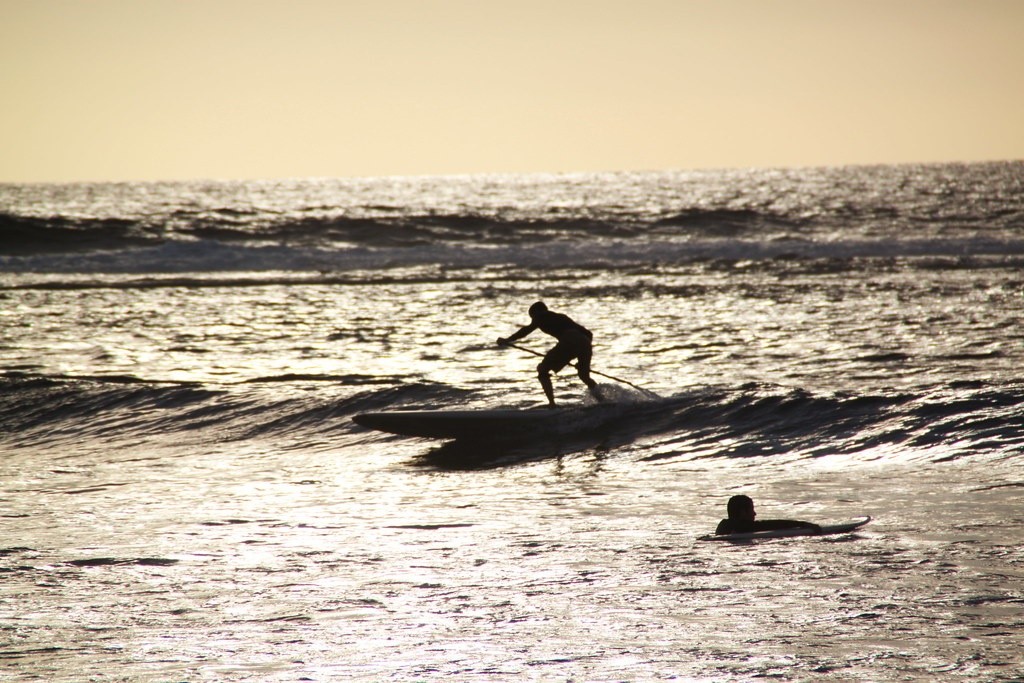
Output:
[715,495,822,536]
[497,302,606,407]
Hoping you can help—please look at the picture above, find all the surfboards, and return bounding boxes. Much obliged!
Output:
[350,402,613,441]
[695,514,871,540]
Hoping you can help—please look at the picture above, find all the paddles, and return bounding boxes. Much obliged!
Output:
[504,341,662,399]
